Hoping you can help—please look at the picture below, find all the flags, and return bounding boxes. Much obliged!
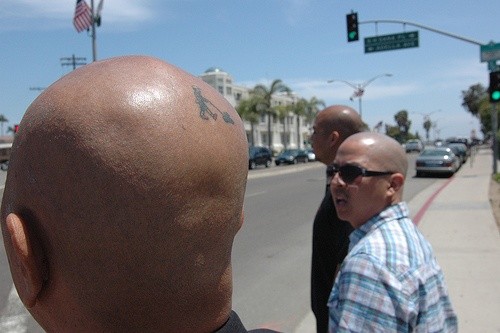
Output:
[73,0,91,33]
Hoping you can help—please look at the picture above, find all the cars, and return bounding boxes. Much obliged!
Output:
[274,148,308,164]
[406,140,424,153]
[414,146,460,177]
[244,145,272,169]
[450,141,469,164]
[304,149,317,161]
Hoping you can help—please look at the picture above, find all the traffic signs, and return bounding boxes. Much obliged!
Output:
[364,30,419,55]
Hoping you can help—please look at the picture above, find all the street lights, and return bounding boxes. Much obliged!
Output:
[408,109,442,143]
[326,74,393,117]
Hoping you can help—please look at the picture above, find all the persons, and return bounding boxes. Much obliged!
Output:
[310,105,364,333]
[0,54,282,333]
[326,132,459,333]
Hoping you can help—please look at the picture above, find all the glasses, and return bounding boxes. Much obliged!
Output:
[326,164,397,182]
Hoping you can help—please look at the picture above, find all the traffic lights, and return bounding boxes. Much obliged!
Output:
[346,12,359,43]
[487,71,500,103]
[15,124,20,136]
